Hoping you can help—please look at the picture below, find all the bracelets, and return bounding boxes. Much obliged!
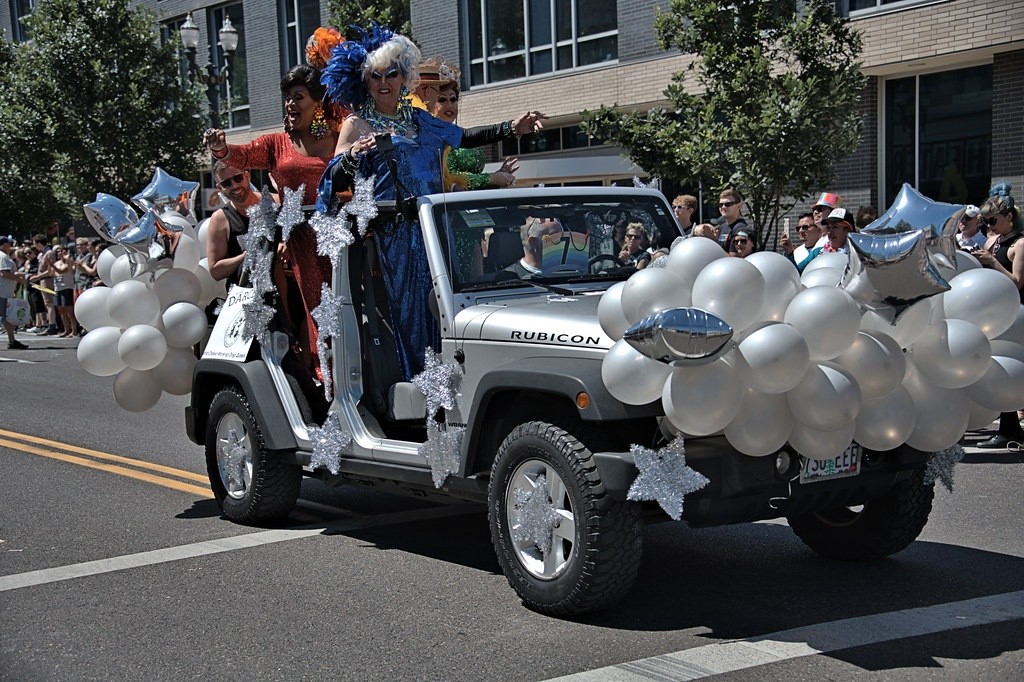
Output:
[503,119,523,140]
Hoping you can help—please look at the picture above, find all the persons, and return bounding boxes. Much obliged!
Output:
[855,204,879,230]
[970,182,1024,447]
[780,206,857,270]
[202,66,342,429]
[811,187,843,253]
[956,204,989,252]
[207,160,282,294]
[316,23,550,444]
[779,192,822,272]
[0,233,110,349]
[672,194,699,238]
[731,225,758,257]
[501,214,669,278]
[715,189,749,257]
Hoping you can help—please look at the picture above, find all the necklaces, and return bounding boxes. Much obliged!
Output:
[993,229,1015,255]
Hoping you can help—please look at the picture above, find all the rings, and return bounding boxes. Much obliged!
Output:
[203,128,216,140]
[511,178,518,186]
[532,110,546,120]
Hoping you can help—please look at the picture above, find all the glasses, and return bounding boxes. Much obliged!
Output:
[77,243,84,246]
[24,252,32,255]
[796,224,816,232]
[672,205,691,211]
[421,85,442,98]
[982,216,998,226]
[961,216,975,221]
[719,202,740,207]
[812,206,827,212]
[218,172,244,188]
[734,239,751,245]
[992,240,1000,258]
[626,234,642,240]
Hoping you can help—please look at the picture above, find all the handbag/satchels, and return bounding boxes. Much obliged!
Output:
[5,268,31,326]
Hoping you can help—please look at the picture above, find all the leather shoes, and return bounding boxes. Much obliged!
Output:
[977,433,1023,448]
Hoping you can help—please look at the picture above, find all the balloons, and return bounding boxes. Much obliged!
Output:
[597,183,1024,448]
[71,163,228,413]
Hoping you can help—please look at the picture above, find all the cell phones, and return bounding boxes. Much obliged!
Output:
[784,218,790,239]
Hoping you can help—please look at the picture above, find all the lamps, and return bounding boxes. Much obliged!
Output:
[491,38,508,65]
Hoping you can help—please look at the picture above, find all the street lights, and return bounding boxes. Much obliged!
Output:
[179,11,239,187]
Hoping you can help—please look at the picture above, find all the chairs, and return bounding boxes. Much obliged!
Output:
[487,232,525,273]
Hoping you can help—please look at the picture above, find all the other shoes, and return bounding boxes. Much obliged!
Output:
[8,340,29,349]
[17,326,58,336]
[60,332,80,338]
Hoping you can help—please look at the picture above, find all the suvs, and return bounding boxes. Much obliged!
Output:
[183,186,938,618]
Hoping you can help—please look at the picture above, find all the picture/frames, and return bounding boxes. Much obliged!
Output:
[891,134,992,184]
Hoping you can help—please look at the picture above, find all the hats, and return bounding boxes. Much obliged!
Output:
[411,64,450,86]
[733,227,752,239]
[821,208,853,225]
[965,205,980,217]
[816,192,843,209]
[0,236,14,246]
